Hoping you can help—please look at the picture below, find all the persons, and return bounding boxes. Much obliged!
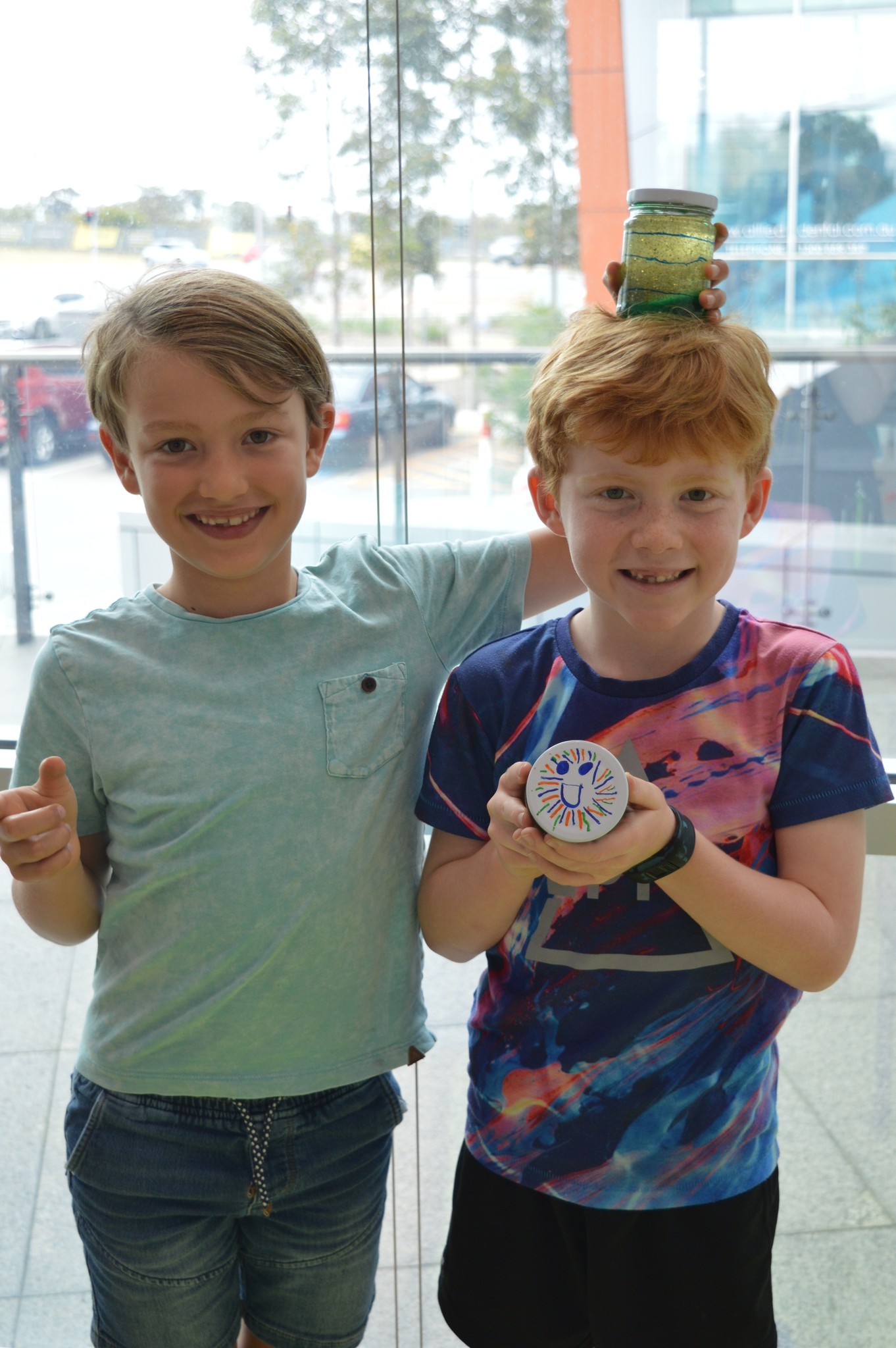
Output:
[0,219,729,1346]
[410,311,896,1348]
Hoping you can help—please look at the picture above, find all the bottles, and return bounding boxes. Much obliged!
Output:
[616,185,718,319]
[526,741,629,844]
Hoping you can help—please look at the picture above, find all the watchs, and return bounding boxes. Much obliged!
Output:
[624,812,692,892]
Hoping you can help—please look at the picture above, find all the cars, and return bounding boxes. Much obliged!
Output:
[1,342,94,468]
[325,361,456,466]
[143,238,196,266]
